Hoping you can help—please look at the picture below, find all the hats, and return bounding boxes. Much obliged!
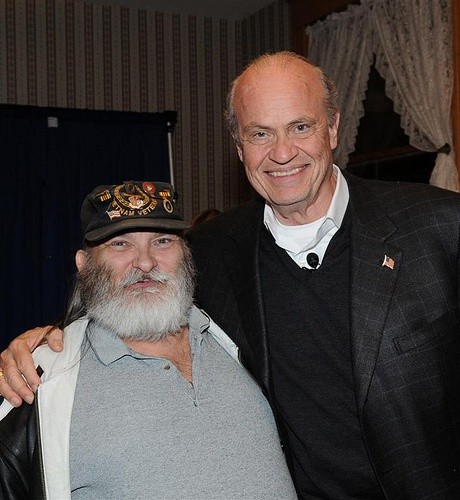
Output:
[76,180,191,235]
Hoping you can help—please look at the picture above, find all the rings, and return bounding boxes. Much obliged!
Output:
[0,370,5,376]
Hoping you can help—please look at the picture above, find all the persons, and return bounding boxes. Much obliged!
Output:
[0,52,460,500]
[0,181,298,500]
